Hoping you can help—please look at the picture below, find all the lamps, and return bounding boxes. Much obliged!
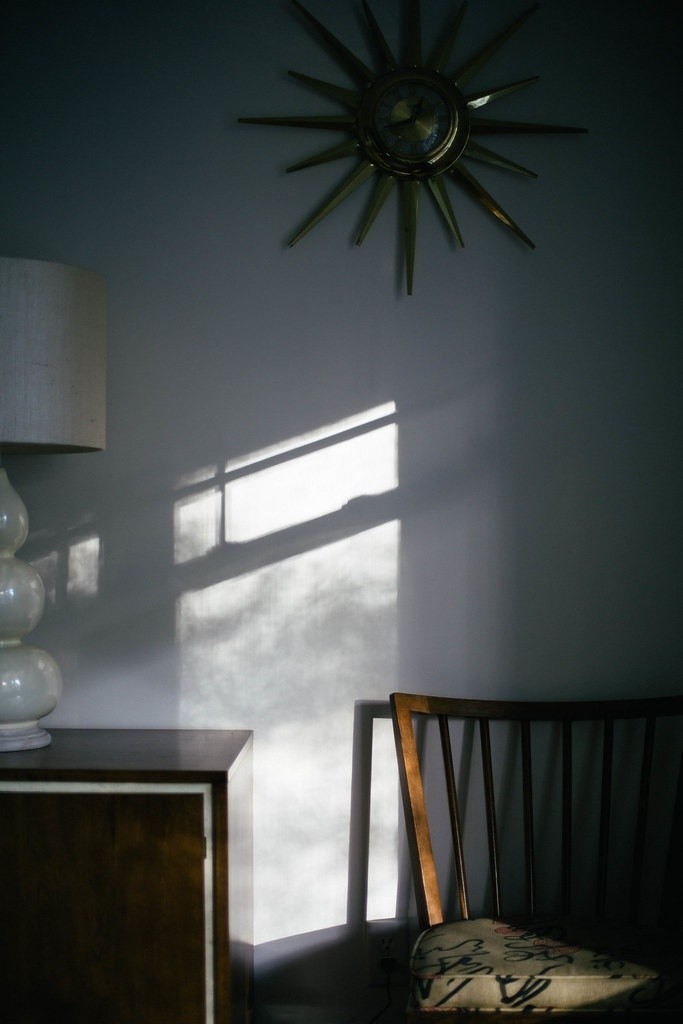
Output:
[0,256,108,752]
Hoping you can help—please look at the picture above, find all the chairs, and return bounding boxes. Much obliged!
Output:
[391,692,683,1024]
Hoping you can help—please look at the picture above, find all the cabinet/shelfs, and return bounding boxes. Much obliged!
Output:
[0,728,256,1024]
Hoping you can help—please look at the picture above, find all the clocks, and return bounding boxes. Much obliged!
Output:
[238,0,589,296]
